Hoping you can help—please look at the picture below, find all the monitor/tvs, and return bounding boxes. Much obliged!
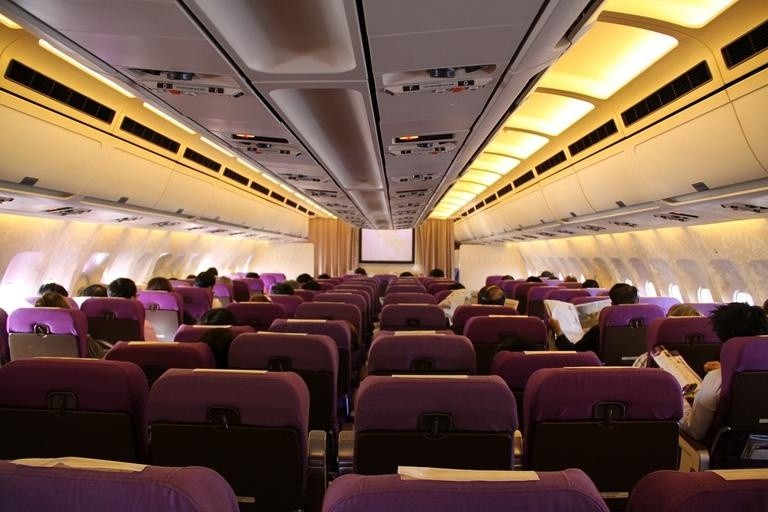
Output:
[357,227,417,264]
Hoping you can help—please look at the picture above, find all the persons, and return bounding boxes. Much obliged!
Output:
[447,283,465,289]
[501,270,768,442]
[401,271,414,276]
[32,269,331,390]
[429,269,444,277]
[439,285,506,323]
[354,268,368,274]
